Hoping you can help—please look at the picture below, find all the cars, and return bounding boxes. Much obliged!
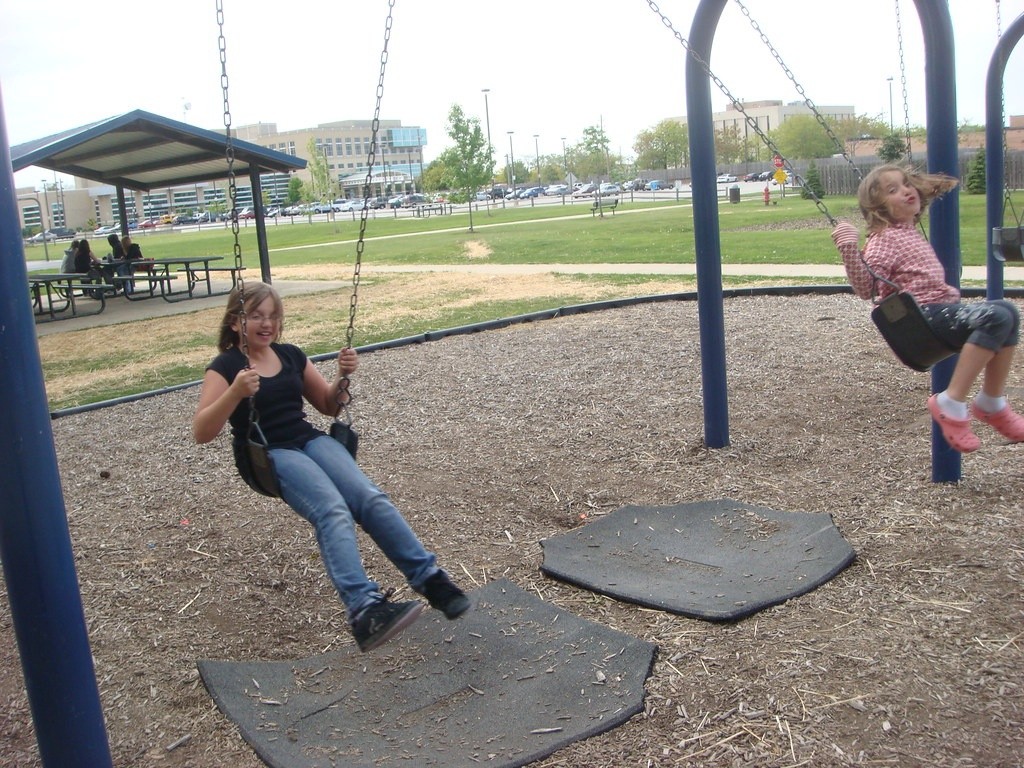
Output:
[389,177,676,209]
[758,172,773,181]
[89,183,386,235]
[717,173,738,183]
[771,173,794,185]
[743,173,761,182]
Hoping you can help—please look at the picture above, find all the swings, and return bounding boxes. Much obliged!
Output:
[645,0,969,375]
[217,0,398,499]
[992,0,1024,263]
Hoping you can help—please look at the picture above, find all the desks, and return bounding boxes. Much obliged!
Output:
[413,204,443,219]
[123,256,236,303]
[29,273,105,324]
[58,258,157,300]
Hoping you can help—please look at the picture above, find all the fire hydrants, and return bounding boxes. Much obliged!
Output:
[762,187,770,206]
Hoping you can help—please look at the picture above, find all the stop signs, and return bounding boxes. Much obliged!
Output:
[774,155,784,167]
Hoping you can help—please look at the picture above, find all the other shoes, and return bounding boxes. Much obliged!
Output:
[107,289,121,295]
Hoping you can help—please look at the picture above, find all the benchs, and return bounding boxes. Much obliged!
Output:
[177,266,246,272]
[424,207,443,211]
[409,209,422,211]
[74,275,98,281]
[53,284,116,290]
[112,275,179,281]
[590,199,620,217]
[135,267,165,271]
[29,284,44,288]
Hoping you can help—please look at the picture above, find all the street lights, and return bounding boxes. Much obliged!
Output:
[885,76,893,134]
[379,145,388,205]
[561,137,567,173]
[322,143,332,207]
[481,89,496,207]
[506,132,517,200]
[533,134,541,194]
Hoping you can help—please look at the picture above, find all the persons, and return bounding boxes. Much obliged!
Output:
[829,164,1024,456]
[192,281,473,653]
[60,239,113,296]
[103,234,126,287]
[120,236,143,295]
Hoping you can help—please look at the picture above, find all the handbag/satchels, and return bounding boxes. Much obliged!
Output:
[137,256,154,270]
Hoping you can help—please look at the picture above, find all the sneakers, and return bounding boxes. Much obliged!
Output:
[352,586,419,652]
[422,568,471,619]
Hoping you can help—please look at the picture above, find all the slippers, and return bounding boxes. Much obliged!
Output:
[928,393,981,453]
[971,395,1024,441]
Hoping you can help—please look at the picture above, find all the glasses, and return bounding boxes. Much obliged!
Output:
[229,310,281,323]
